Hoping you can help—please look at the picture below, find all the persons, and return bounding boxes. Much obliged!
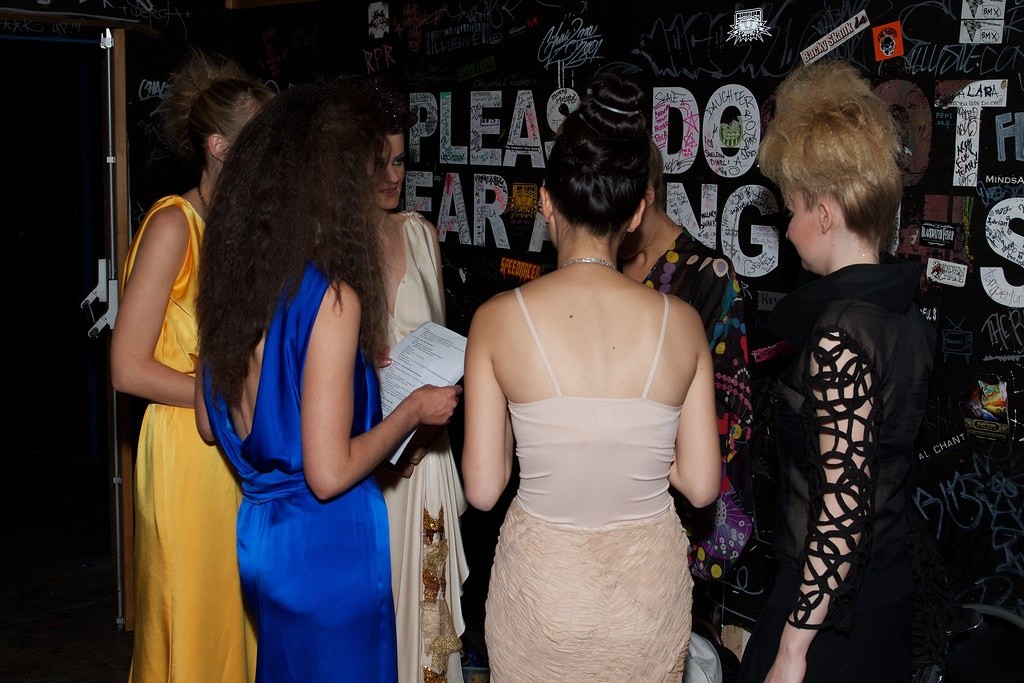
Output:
[111,49,937,683]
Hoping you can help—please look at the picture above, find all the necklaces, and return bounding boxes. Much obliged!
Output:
[560,258,616,270]
[196,185,209,218]
